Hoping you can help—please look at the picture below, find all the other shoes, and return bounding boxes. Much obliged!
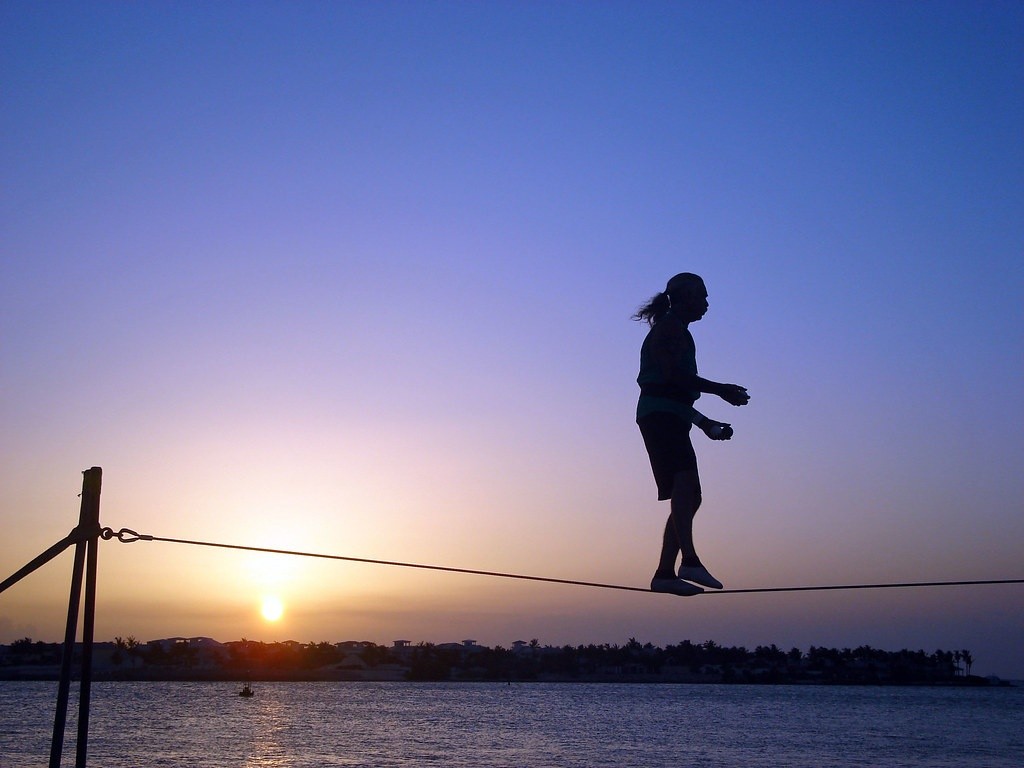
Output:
[650,576,703,596]
[678,565,723,589]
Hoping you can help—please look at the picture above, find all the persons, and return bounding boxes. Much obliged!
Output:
[630,272,750,597]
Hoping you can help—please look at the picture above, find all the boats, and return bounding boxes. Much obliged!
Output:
[239,683,255,698]
